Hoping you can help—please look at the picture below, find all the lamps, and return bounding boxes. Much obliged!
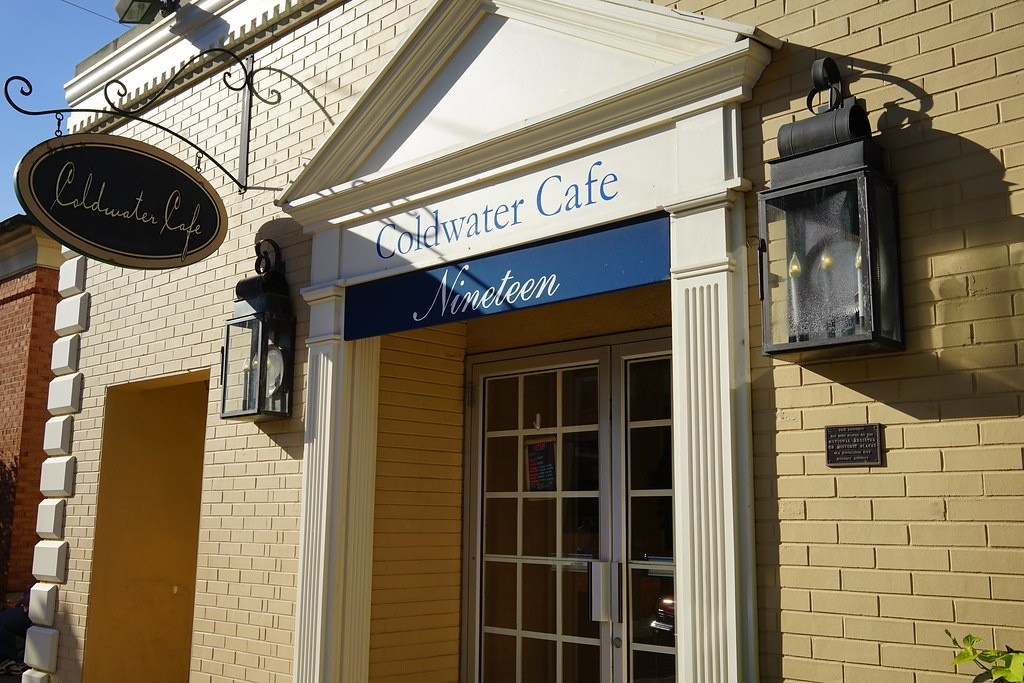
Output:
[114,0,182,25]
[747,56,908,365]
[219,238,297,424]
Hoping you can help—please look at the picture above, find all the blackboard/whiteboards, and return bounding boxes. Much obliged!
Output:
[525,437,558,501]
